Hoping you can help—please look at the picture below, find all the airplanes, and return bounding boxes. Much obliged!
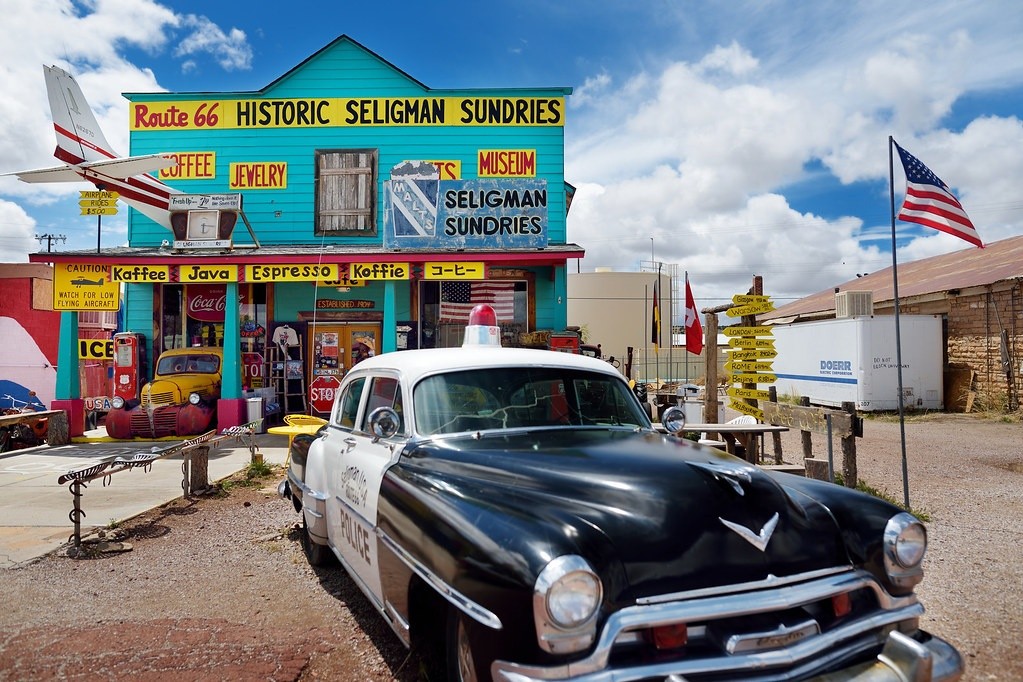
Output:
[0,61,193,235]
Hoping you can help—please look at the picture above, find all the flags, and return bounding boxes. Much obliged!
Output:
[652,289,658,352]
[892,140,985,249]
[685,281,702,355]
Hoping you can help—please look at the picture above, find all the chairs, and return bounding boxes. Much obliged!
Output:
[281,414,329,476]
[717,377,728,396]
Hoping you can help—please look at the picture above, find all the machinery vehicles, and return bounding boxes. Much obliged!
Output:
[578,344,651,426]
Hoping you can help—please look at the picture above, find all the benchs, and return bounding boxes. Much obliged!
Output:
[700,442,746,461]
[756,465,805,470]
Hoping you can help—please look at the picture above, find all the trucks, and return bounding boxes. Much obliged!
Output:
[105,346,246,438]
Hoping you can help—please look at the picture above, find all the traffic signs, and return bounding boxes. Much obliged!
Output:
[722,293,778,422]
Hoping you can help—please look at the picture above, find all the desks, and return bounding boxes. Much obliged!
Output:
[267,425,324,479]
[651,423,789,466]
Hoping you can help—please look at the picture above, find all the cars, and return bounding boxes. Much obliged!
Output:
[279,303,964,680]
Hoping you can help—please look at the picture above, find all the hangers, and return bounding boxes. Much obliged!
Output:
[283,322,290,329]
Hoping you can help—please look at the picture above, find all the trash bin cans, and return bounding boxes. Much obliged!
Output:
[245,397,266,434]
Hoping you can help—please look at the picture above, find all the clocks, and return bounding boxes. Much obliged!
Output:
[187,209,220,240]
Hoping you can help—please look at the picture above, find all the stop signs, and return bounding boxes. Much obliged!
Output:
[308,375,340,414]
[241,351,265,389]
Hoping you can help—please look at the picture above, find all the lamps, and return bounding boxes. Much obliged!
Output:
[159,239,169,251]
